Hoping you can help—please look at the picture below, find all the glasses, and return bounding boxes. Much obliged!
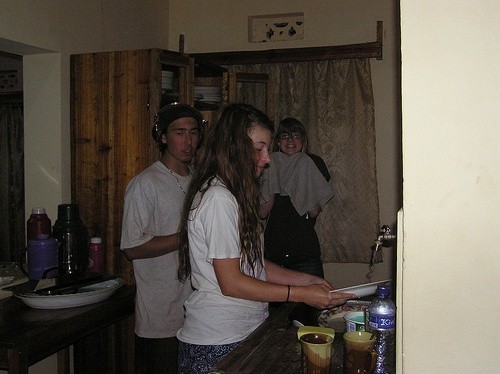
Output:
[278,130,302,140]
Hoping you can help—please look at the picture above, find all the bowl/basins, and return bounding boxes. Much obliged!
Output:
[161,70,175,96]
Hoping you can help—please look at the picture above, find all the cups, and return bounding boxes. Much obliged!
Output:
[343,332,377,374]
[342,311,365,332]
[300,333,334,374]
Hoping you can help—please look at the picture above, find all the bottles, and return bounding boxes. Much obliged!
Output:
[364,285,396,374]
[88,237,105,274]
[27,234,59,280]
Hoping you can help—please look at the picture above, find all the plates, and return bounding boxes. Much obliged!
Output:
[318,300,372,333]
[9,279,123,309]
[194,85,222,105]
[328,280,393,298]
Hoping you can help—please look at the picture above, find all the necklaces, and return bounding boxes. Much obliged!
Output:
[161,160,194,197]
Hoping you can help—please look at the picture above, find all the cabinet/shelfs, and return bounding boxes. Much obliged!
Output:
[69,49,227,374]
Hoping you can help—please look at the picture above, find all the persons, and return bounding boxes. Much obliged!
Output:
[257,118,331,315]
[177,103,357,374]
[119,103,205,374]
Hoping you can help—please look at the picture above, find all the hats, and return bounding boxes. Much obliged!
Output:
[151,101,205,143]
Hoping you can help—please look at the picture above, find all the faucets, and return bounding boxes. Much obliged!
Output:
[373,225,396,252]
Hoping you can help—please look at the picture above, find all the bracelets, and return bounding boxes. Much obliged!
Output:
[286,285,290,302]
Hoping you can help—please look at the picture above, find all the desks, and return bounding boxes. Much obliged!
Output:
[0,287,136,374]
[209,303,345,374]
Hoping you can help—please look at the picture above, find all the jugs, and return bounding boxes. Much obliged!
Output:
[52,203,88,282]
[27,207,52,251]
